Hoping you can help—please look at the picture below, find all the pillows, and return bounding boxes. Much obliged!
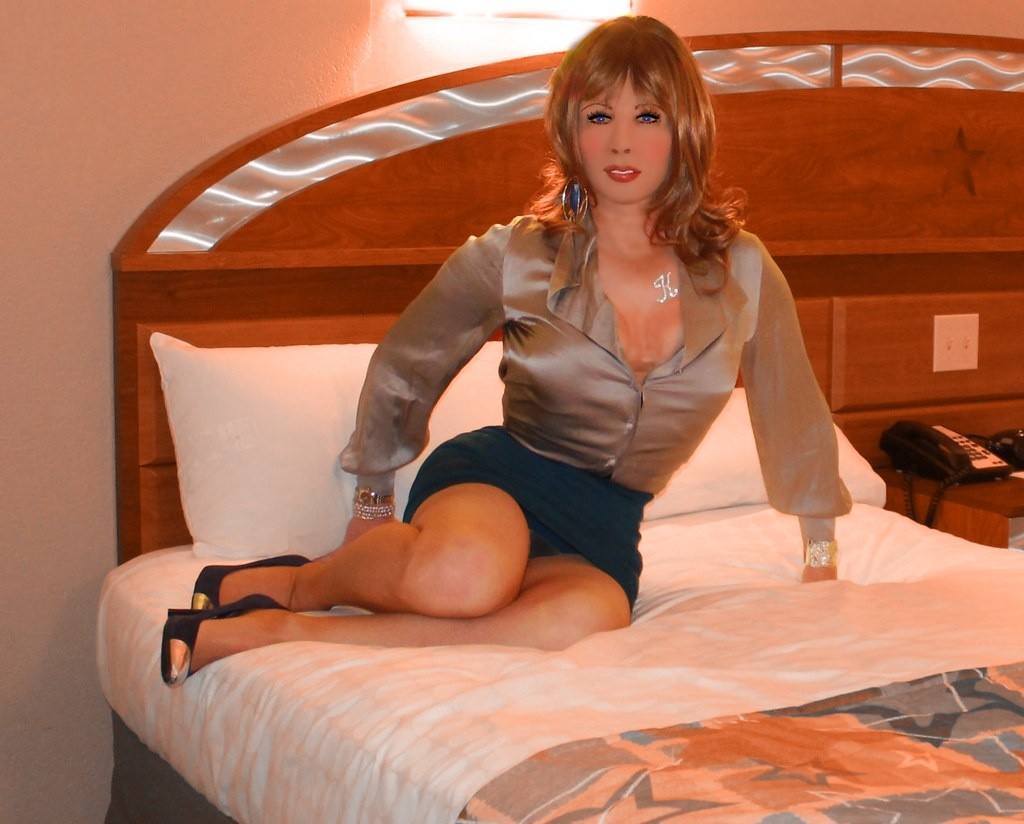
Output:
[147,332,886,560]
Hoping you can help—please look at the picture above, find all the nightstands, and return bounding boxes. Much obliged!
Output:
[874,468,1024,548]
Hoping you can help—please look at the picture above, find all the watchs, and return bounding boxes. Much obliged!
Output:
[354,488,395,505]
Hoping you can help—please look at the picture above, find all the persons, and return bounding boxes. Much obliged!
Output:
[161,17,852,687]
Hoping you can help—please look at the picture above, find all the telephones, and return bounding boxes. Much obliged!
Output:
[878,419,1014,487]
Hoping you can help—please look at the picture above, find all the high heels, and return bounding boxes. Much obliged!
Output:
[191,554,314,610]
[160,608,243,688]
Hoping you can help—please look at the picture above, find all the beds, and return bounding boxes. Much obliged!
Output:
[97,26,1024,824]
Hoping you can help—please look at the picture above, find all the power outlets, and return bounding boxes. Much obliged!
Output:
[930,313,979,373]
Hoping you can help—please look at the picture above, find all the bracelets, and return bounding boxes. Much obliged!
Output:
[352,503,395,519]
[805,539,839,568]
